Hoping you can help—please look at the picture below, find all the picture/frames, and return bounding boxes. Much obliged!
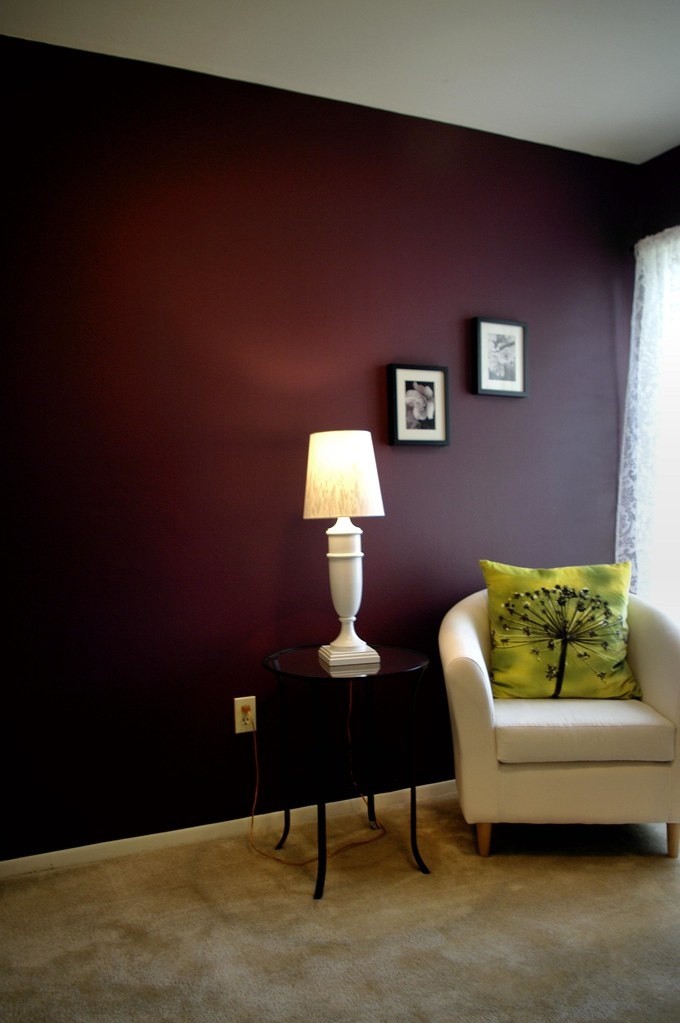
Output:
[471,317,529,398]
[391,364,451,447]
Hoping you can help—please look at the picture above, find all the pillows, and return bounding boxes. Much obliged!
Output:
[482,560,644,700]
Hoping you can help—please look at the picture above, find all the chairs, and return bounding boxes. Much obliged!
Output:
[438,589,680,860]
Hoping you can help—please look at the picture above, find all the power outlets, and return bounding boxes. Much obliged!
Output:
[234,694,256,735]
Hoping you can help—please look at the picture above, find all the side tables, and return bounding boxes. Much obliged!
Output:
[262,643,428,901]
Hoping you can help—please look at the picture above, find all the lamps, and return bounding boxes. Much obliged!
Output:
[301,430,386,666]
